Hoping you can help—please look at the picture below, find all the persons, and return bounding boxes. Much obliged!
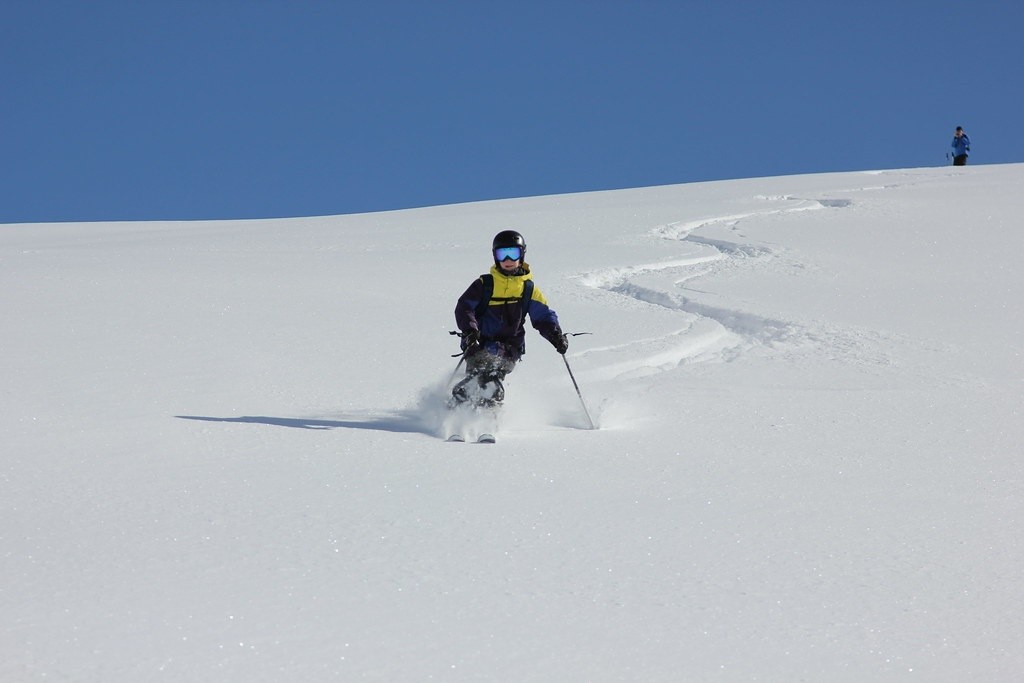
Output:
[952,126,970,166]
[446,230,569,442]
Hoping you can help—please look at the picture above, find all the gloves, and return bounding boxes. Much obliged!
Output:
[556,334,568,354]
[465,328,480,350]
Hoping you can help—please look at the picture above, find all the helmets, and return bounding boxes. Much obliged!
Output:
[492,230,526,267]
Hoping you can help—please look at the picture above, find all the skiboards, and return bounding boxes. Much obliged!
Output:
[446,431,496,445]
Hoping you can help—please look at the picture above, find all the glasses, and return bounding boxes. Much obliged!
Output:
[495,247,522,261]
